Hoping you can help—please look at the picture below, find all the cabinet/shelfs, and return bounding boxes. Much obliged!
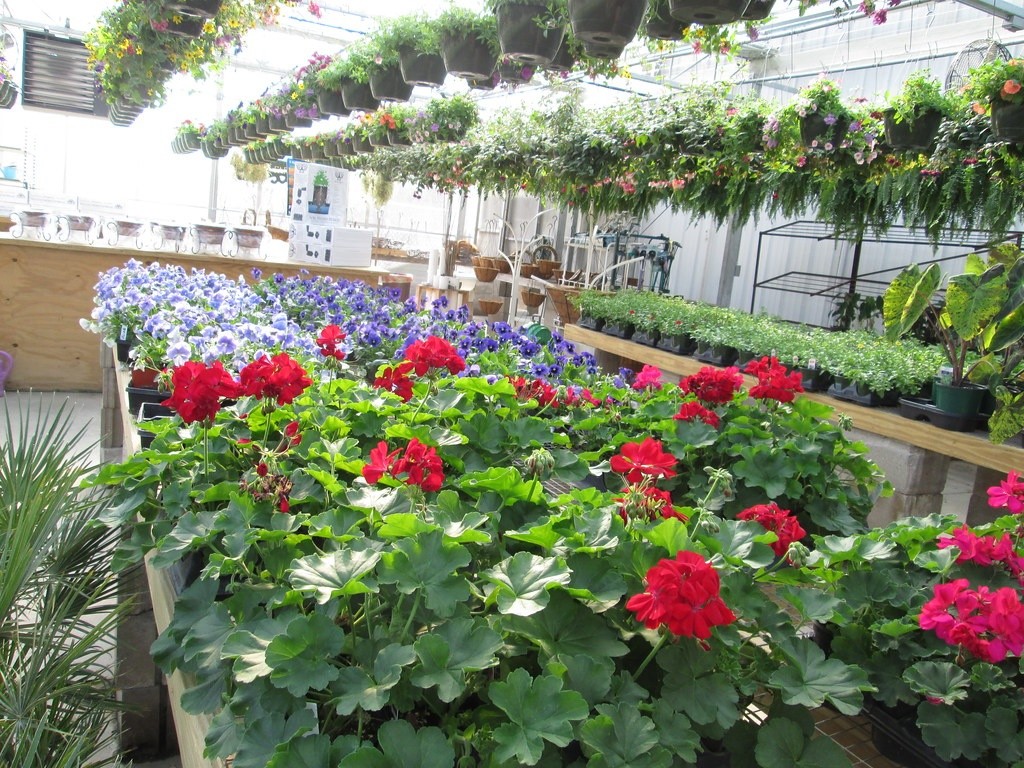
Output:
[749,220,1024,331]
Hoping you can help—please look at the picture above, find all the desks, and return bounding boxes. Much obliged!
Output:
[416,284,469,310]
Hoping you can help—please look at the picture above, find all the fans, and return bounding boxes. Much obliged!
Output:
[944,38,1012,88]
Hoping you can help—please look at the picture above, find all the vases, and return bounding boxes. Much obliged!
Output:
[124,378,172,414]
[988,94,1024,143]
[232,226,265,250]
[466,67,499,91]
[498,64,538,85]
[129,362,167,389]
[645,13,690,41]
[796,112,854,150]
[539,33,575,71]
[883,107,942,151]
[492,3,565,65]
[439,22,499,81]
[136,401,186,459]
[601,317,635,339]
[655,333,696,356]
[675,125,724,156]
[116,219,144,237]
[583,41,624,59]
[631,325,660,346]
[667,0,750,25]
[567,0,648,47]
[114,337,137,366]
[812,620,869,714]
[860,693,981,768]
[66,215,94,232]
[192,223,226,244]
[21,210,51,228]
[158,224,187,240]
[105,0,465,166]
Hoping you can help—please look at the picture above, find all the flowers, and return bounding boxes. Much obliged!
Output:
[647,40,677,55]
[808,469,1024,768]
[743,15,778,43]
[78,259,896,768]
[339,55,1024,258]
[0,0,338,153]
[856,0,901,26]
[682,24,742,62]
[782,0,852,18]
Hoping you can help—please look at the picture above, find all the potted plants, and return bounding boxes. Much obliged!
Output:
[882,242,1024,414]
[565,288,611,330]
[768,338,828,392]
[689,319,736,367]
[731,327,772,377]
[819,347,899,409]
